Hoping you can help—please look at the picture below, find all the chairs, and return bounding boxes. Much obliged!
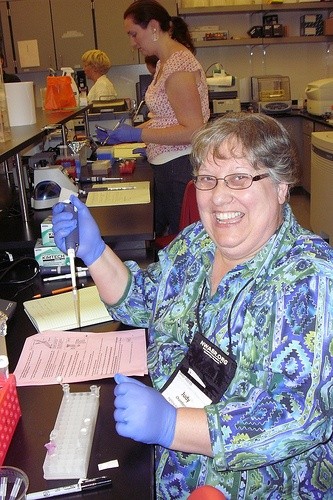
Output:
[155,181,199,249]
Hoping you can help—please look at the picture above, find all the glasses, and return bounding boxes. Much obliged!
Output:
[191,174,270,190]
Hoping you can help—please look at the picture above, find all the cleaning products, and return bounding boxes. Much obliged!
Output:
[60,66,80,110]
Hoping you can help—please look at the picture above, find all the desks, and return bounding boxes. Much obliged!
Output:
[0,249,156,500]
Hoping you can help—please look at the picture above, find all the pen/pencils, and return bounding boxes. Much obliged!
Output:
[26,476,111,500]
[9,478,23,500]
[32,282,85,299]
[102,114,129,145]
[0,477,8,500]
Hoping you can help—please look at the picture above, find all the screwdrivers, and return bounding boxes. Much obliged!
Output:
[62,203,80,323]
[84,186,137,192]
[43,270,92,282]
[75,176,124,182]
[40,266,88,275]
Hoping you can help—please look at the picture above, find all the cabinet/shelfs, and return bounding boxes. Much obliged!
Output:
[0,0,177,74]
[303,118,333,194]
[178,1,333,48]
[0,105,154,249]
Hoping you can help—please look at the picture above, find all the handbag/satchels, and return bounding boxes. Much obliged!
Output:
[43,76,77,111]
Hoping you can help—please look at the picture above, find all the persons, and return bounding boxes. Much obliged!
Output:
[0,53,21,83]
[81,49,117,100]
[51,112,333,500]
[96,0,210,262]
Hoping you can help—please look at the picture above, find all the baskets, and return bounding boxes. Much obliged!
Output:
[0,373,23,468]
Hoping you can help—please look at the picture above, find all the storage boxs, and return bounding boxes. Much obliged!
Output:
[33,213,71,265]
[190,15,333,40]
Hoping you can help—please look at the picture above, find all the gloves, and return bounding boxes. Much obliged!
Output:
[106,124,142,142]
[51,195,105,266]
[113,374,175,449]
[96,128,123,144]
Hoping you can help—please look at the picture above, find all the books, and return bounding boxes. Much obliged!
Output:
[23,285,113,333]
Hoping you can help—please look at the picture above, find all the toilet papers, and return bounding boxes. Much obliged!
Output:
[4,80,37,127]
